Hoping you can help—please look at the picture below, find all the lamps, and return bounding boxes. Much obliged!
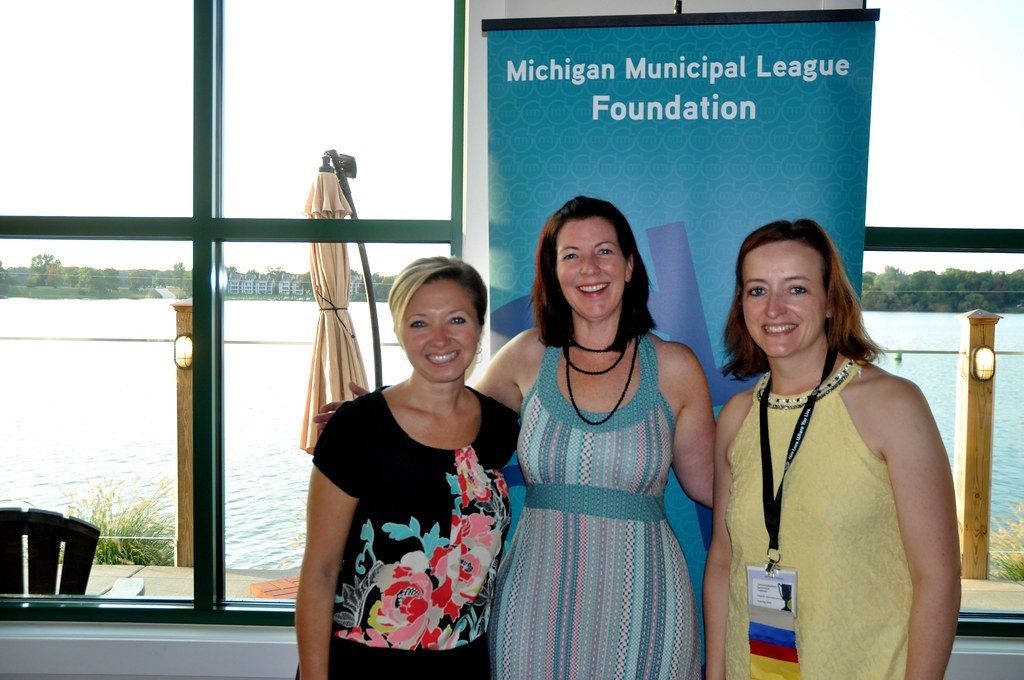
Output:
[174,334,193,369]
[970,345,996,380]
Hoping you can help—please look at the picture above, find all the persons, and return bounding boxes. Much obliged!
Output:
[296,254,520,680]
[703,217,962,680]
[314,195,715,680]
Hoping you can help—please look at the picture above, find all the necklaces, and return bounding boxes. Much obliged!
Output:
[563,334,639,426]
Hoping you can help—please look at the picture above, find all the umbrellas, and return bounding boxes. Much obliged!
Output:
[302,150,387,456]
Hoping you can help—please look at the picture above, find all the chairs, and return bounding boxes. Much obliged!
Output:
[0,508,145,597]
